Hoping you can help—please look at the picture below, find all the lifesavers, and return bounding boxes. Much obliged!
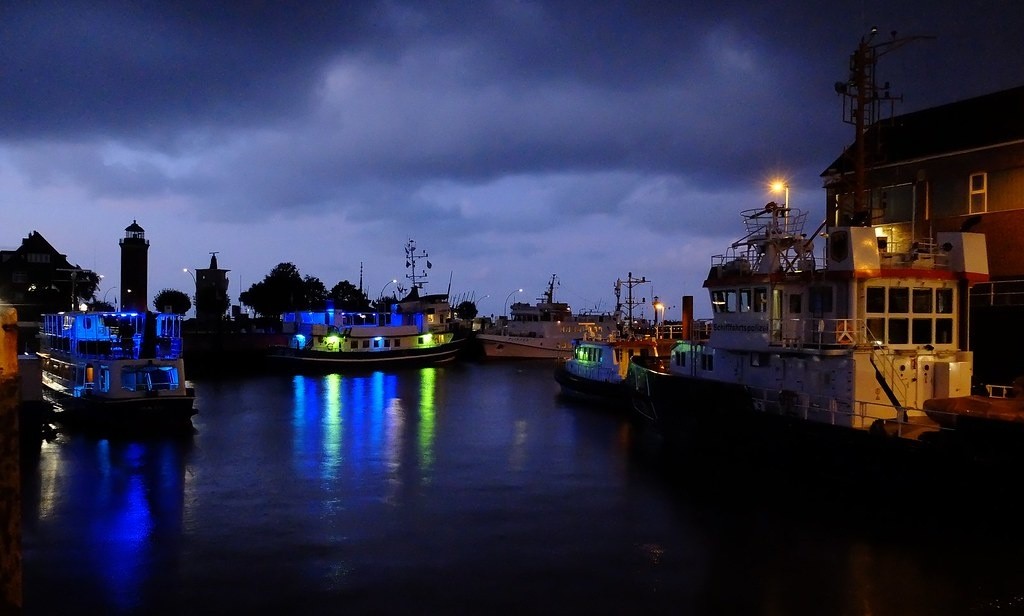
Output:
[836,323,854,343]
[327,343,333,350]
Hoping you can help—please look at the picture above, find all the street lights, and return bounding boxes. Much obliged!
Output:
[654,301,661,339]
[772,182,789,237]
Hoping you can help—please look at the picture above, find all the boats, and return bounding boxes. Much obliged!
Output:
[629,202,1023,525]
[36,310,200,411]
[268,301,466,368]
[468,273,624,357]
[554,338,664,400]
